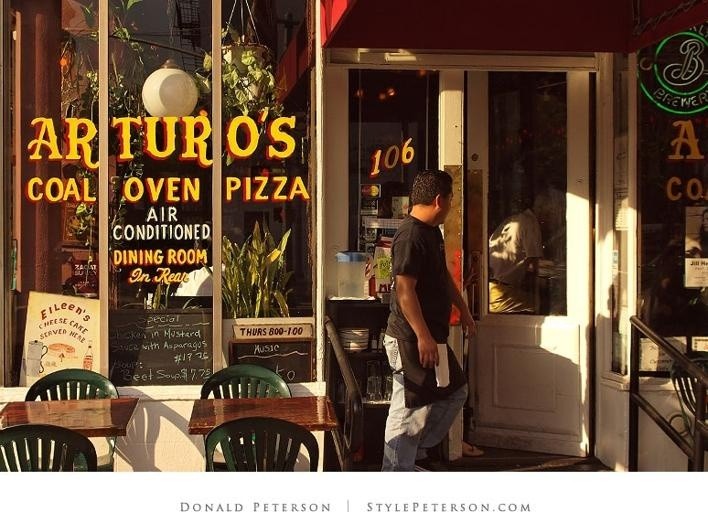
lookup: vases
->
[337,326,370,353]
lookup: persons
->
[460,266,487,455]
[381,169,479,471]
[487,185,567,316]
[172,223,229,298]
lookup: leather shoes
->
[462,446,484,456]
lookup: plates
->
[337,327,370,353]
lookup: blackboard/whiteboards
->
[229,337,317,384]
[109,308,213,387]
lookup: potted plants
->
[194,0,287,117]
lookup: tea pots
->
[22,339,48,376]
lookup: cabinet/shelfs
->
[322,296,469,471]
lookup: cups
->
[336,251,373,298]
[366,376,382,401]
[383,376,392,401]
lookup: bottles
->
[371,327,385,350]
[83,345,93,371]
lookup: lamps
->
[142,1,199,123]
